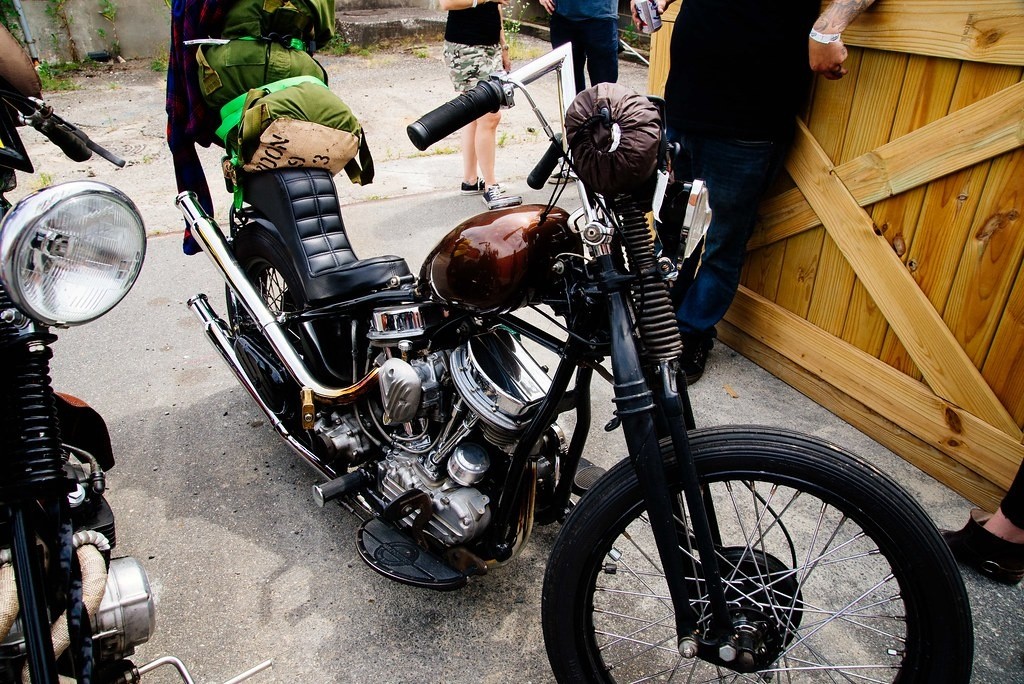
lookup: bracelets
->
[502,44,509,50]
[809,27,841,44]
[472,0,478,8]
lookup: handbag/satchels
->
[536,81,662,228]
[196,0,375,210]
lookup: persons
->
[938,458,1024,586]
[628,0,872,385]
[539,0,619,185]
[438,0,523,210]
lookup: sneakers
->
[460,176,487,196]
[679,326,718,386]
[481,182,522,208]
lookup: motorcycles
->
[0,82,276,684]
[175,37,977,684]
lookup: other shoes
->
[937,507,1024,587]
[548,170,575,184]
